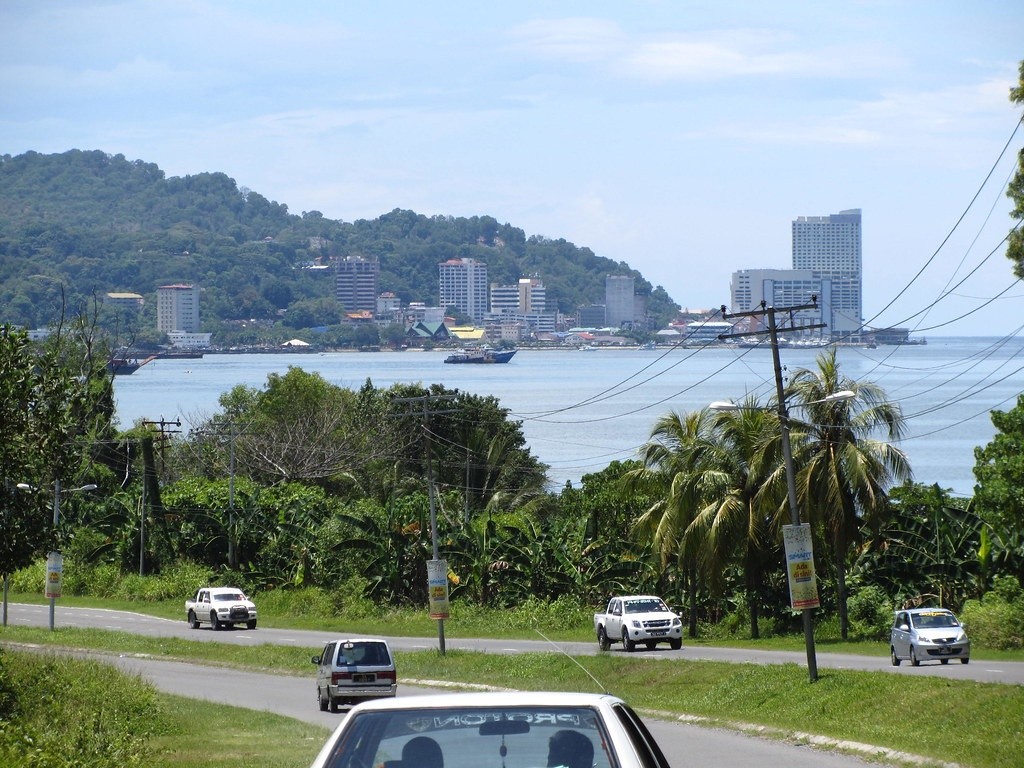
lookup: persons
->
[547,730,594,768]
[401,736,443,768]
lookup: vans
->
[311,639,396,712]
[890,608,971,666]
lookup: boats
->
[109,356,157,375]
[444,349,521,363]
[868,341,876,349]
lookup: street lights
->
[16,482,98,631]
[708,390,856,683]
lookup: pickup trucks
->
[186,587,257,630]
[594,595,682,651]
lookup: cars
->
[308,691,669,768]
